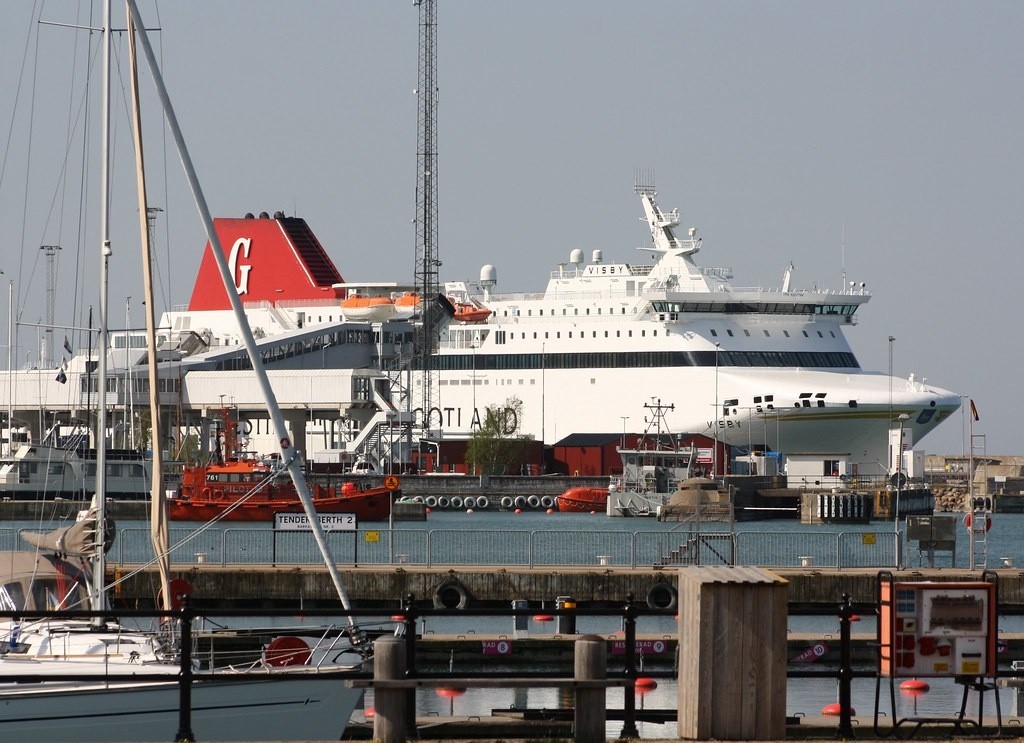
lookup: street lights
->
[713,340,720,476]
[469,343,475,471]
[890,412,911,568]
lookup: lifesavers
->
[413,495,424,505]
[637,580,680,616]
[542,495,554,509]
[476,495,491,510]
[515,494,526,511]
[396,494,412,503]
[197,486,214,501]
[965,512,995,536]
[211,488,227,502]
[500,494,515,511]
[555,495,564,508]
[438,495,450,510]
[527,494,540,509]
[425,494,438,509]
[450,495,464,509]
[462,495,476,510]
[426,580,472,612]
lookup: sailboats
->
[0,1,374,741]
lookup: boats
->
[452,302,493,321]
[170,394,401,523]
[0,167,970,489]
[607,397,707,517]
[0,276,194,499]
